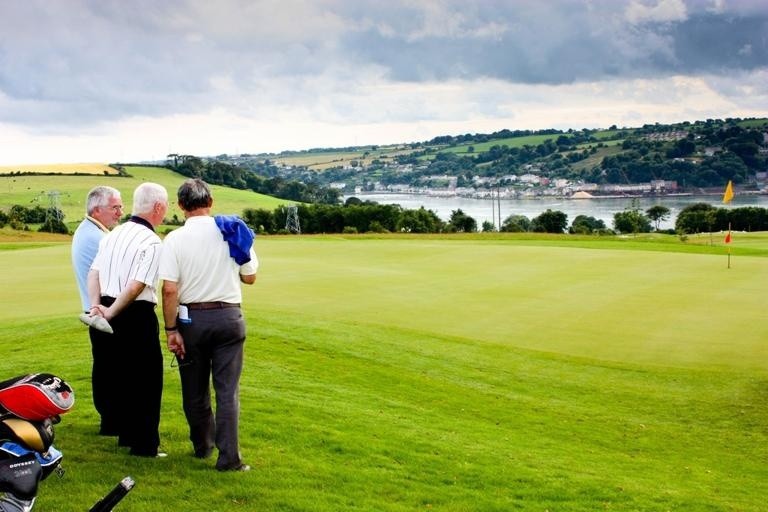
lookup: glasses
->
[171,351,194,368]
[105,206,124,211]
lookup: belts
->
[189,302,240,309]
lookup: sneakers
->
[227,464,251,471]
[155,450,167,457]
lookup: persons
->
[160,178,259,472]
[70,186,126,438]
[86,181,173,459]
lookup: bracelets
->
[164,325,177,331]
